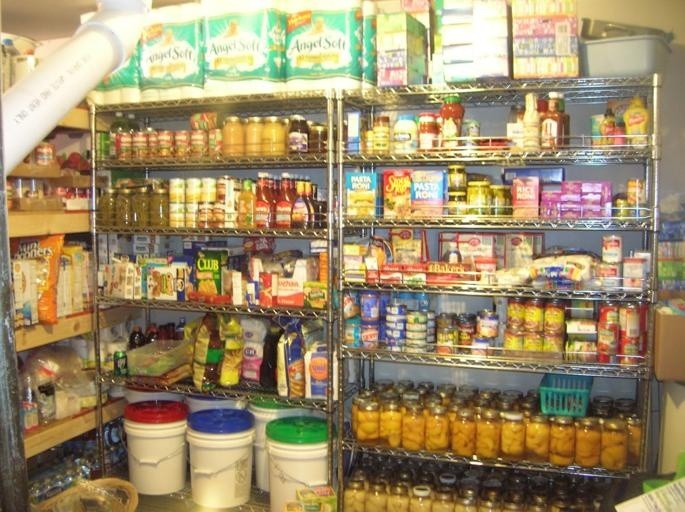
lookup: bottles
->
[366,89,649,151]
[128,318,187,351]
[109,109,140,158]
[238,171,321,229]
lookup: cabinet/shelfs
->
[335,72,667,511]
[0,90,141,466]
[80,86,363,511]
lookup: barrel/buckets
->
[184,393,248,414]
[124,387,186,404]
[246,396,322,493]
[123,398,191,497]
[264,415,337,512]
[186,408,255,509]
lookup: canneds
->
[626,178,644,205]
[7,142,99,200]
[440,96,465,148]
[564,299,598,362]
[115,128,223,160]
[344,291,389,349]
[98,185,170,228]
[345,455,606,512]
[462,119,480,146]
[436,308,500,356]
[190,112,224,131]
[169,175,241,229]
[350,381,642,469]
[506,105,525,154]
[597,301,647,367]
[502,296,566,355]
[418,113,443,149]
[114,350,128,378]
[447,164,510,216]
[222,115,337,157]
[595,262,622,288]
[341,234,651,512]
[385,291,435,353]
[601,236,625,263]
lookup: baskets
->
[539,374,594,417]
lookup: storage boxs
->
[582,32,675,76]
[651,285,684,388]
[648,375,685,479]
